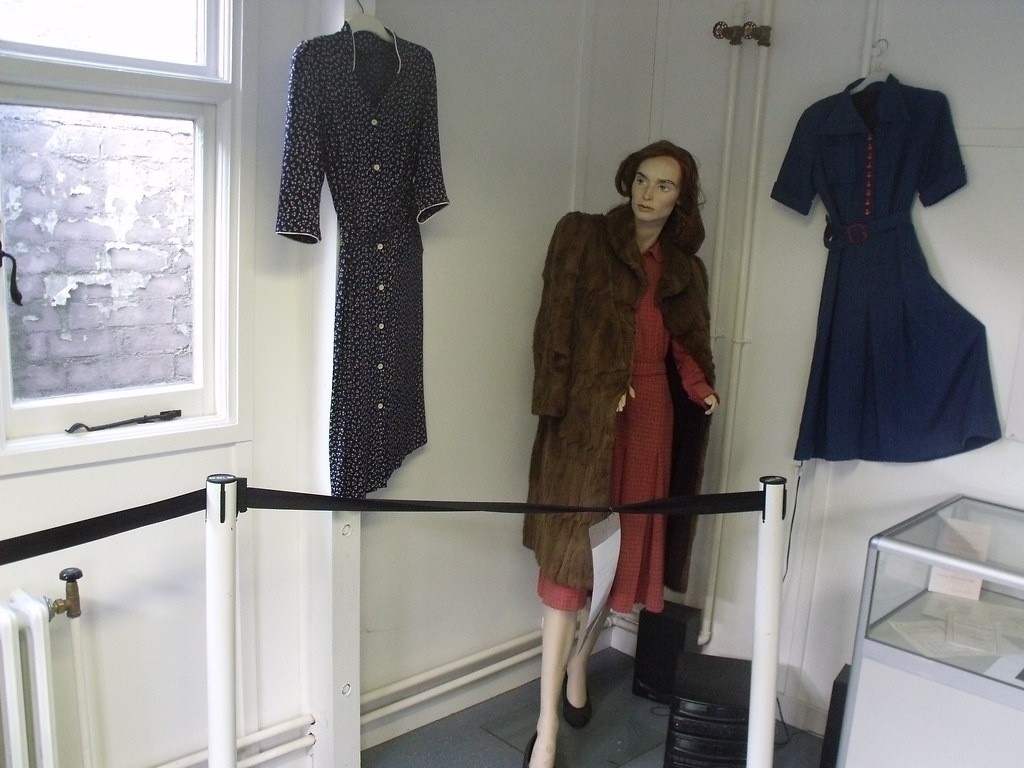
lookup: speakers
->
[632,601,702,704]
[663,652,751,768]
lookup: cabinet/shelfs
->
[838,494,1023,768]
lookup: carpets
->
[480,657,759,768]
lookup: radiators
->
[0,564,80,768]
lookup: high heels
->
[523,731,537,768]
[562,670,593,729]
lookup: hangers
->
[848,39,892,94]
[346,0,395,44]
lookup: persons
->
[523,138,720,768]
